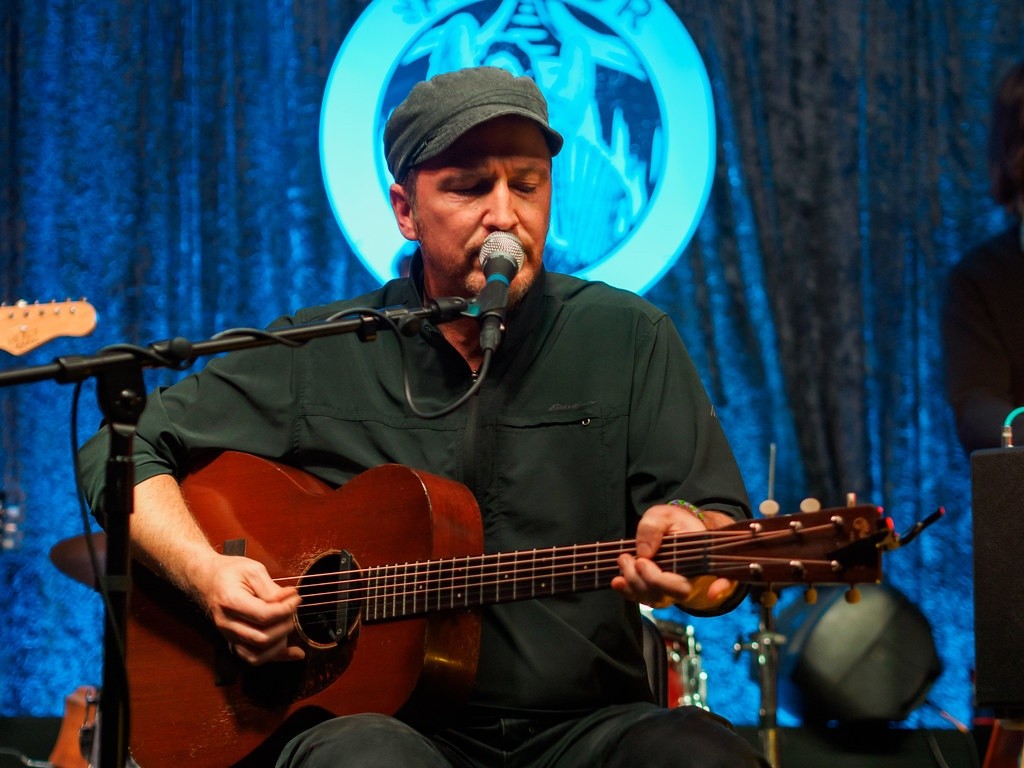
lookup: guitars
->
[0,295,100,359]
[123,447,946,768]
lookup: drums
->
[654,618,705,711]
[772,580,939,725]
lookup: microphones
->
[478,231,524,351]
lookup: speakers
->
[970,447,1024,722]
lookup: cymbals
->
[50,530,109,593]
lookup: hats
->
[384,66,564,185]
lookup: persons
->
[942,60,1024,455]
[71,65,773,768]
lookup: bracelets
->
[669,499,713,529]
